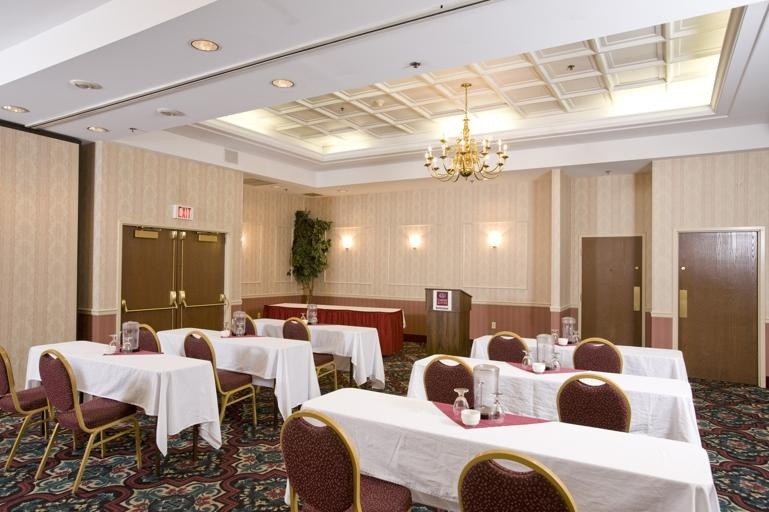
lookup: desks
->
[27,338,223,468]
[159,326,322,432]
[300,385,734,510]
[473,330,693,380]
[404,352,713,440]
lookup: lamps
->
[424,81,510,186]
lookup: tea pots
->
[232,311,247,337]
[561,317,576,344]
[306,303,317,325]
[473,364,499,420]
[536,333,554,368]
[121,320,139,352]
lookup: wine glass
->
[300,312,307,320]
[221,321,231,338]
[108,334,120,353]
[549,352,560,370]
[552,329,559,344]
[235,322,245,337]
[572,330,580,345]
[453,388,469,420]
[522,349,534,370]
[121,337,132,353]
[488,392,505,424]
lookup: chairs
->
[555,372,633,433]
[573,337,624,376]
[457,453,580,512]
[228,317,258,334]
[0,349,52,473]
[181,330,260,440]
[115,323,161,355]
[278,411,411,512]
[280,317,339,394]
[419,356,474,407]
[486,329,529,364]
[35,351,143,495]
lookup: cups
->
[461,410,480,426]
[531,362,545,373]
[557,337,568,346]
